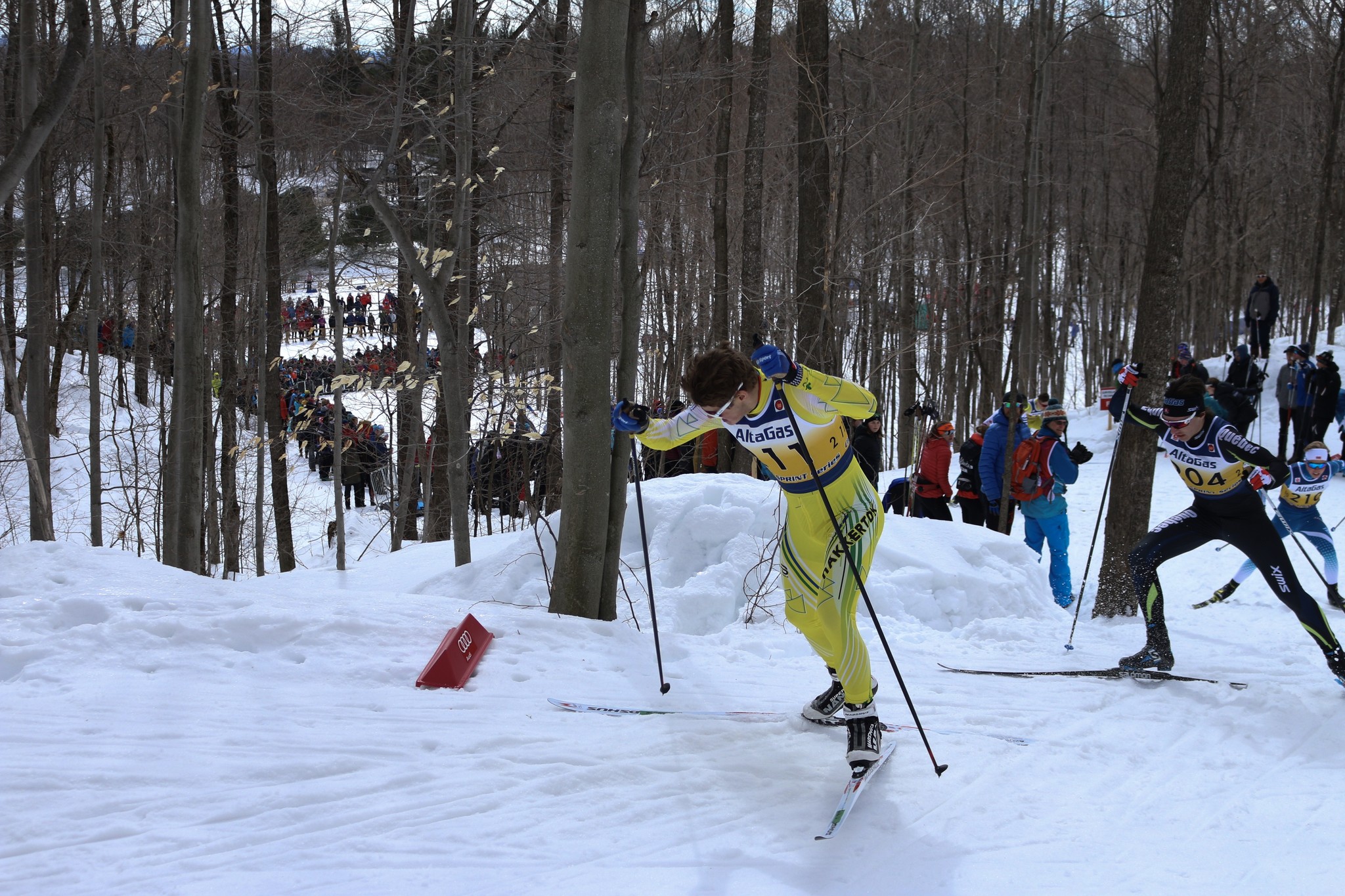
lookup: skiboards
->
[1191,593,1345,610]
[545,691,1030,841]
[934,657,1345,690]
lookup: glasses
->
[1053,419,1068,427]
[691,380,743,419]
[872,413,881,418]
[939,429,957,434]
[1177,346,1190,350]
[1204,383,1213,389]
[1159,410,1196,428]
[1178,350,1191,362]
[1294,349,1300,353]
[1003,401,1022,408]
[1306,459,1326,468]
[1233,353,1240,358]
[1256,274,1266,278]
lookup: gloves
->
[945,495,950,503]
[1117,365,1138,386]
[1269,317,1275,325]
[1249,465,1272,491]
[988,497,1003,513]
[612,400,650,434]
[1071,442,1094,463]
[1244,319,1249,326]
[751,345,798,384]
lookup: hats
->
[1294,343,1312,357]
[865,411,882,426]
[1317,349,1335,364]
[1255,269,1268,275]
[1005,391,1024,410]
[1283,346,1296,353]
[1042,398,1068,433]
[1179,342,1190,349]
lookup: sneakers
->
[1119,645,1177,673]
[1325,642,1345,679]
[1327,585,1345,606]
[1213,579,1239,600]
[807,676,881,729]
[841,699,886,766]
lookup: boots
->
[1275,444,1287,462]
[1287,444,1304,466]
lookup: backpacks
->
[1214,380,1258,425]
[1010,431,1059,500]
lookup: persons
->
[948,407,1000,528]
[1242,269,1280,360]
[881,477,923,519]
[819,275,1086,348]
[1215,441,1345,615]
[7,265,778,520]
[1011,399,1094,608]
[1110,341,1345,477]
[977,390,1032,536]
[841,414,863,441]
[1108,361,1345,691]
[610,342,886,763]
[1021,392,1051,435]
[853,414,886,493]
[911,420,955,522]
